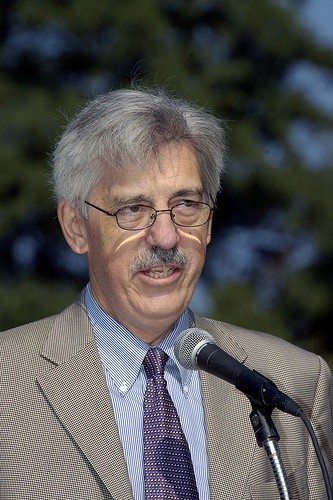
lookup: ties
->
[142,346,200,500]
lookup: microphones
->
[174,327,303,417]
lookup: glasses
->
[77,192,217,231]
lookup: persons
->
[0,85,333,500]
